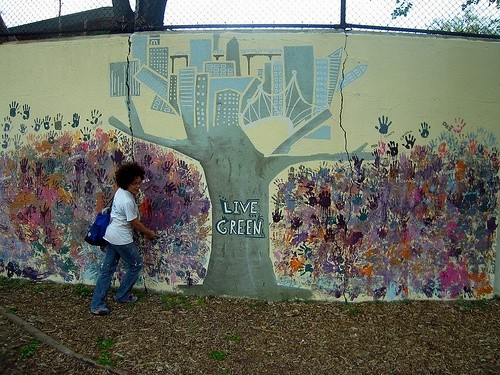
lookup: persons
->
[90,162,161,315]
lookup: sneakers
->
[93,305,111,315]
[119,296,139,303]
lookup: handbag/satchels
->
[85,196,115,247]
